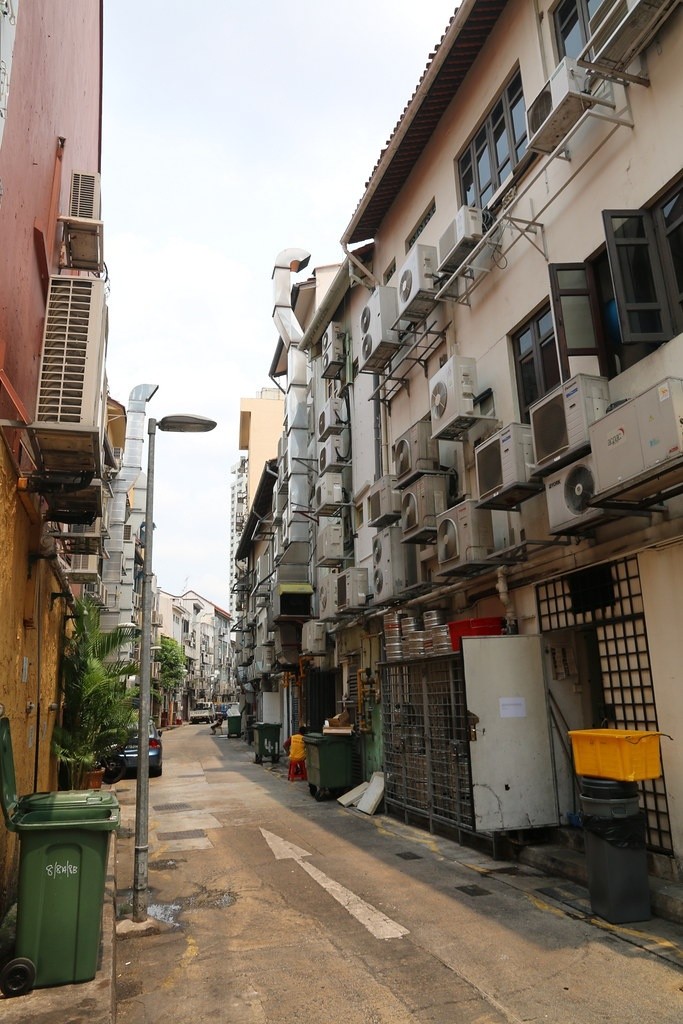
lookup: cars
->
[101,718,164,778]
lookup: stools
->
[288,760,306,781]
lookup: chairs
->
[214,723,223,735]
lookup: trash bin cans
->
[576,774,653,926]
[250,721,282,764]
[226,714,242,740]
[301,732,355,803]
[0,786,121,998]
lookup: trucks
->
[189,700,217,724]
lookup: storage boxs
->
[567,728,662,782]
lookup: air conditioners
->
[32,171,164,675]
[398,244,439,320]
[395,419,438,480]
[371,525,406,601]
[539,453,632,535]
[527,372,613,476]
[335,566,365,614]
[524,56,590,154]
[400,474,448,541]
[428,355,481,440]
[586,376,679,500]
[362,474,402,528]
[588,0,666,65]
[359,286,401,369]
[435,206,484,275]
[435,499,495,577]
[237,322,344,672]
[474,422,545,506]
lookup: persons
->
[211,715,223,735]
[283,725,309,775]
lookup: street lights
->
[132,411,218,925]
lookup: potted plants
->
[52,588,140,791]
